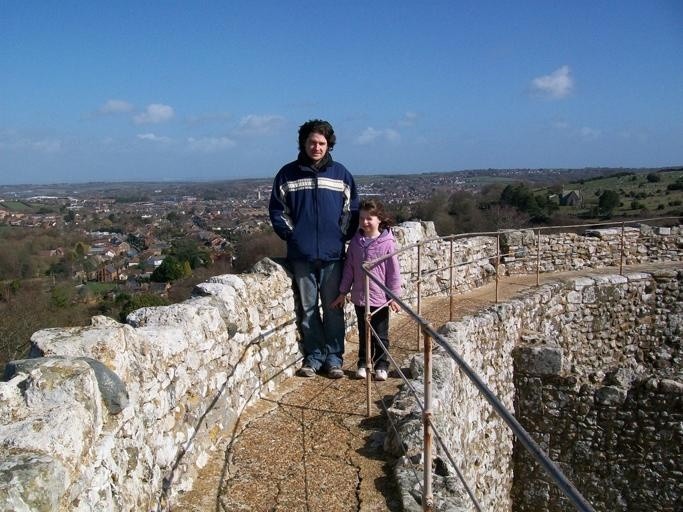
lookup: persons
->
[267,117,362,379]
[328,196,404,382]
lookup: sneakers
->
[326,362,344,379]
[354,366,367,378]
[374,369,389,381]
[298,361,320,376]
